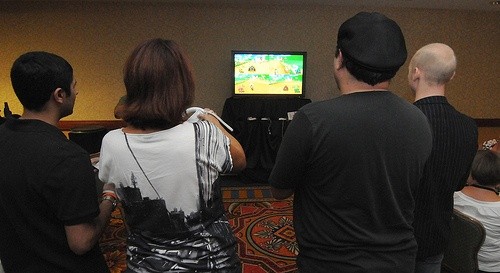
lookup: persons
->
[268,12,432,273]
[454,148,500,273]
[0,52,117,273]
[407,43,479,273]
[98,39,246,273]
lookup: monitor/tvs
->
[230,50,307,98]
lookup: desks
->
[222,96,314,181]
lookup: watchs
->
[100,195,117,211]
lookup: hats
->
[337,11,407,72]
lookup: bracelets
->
[101,191,118,199]
